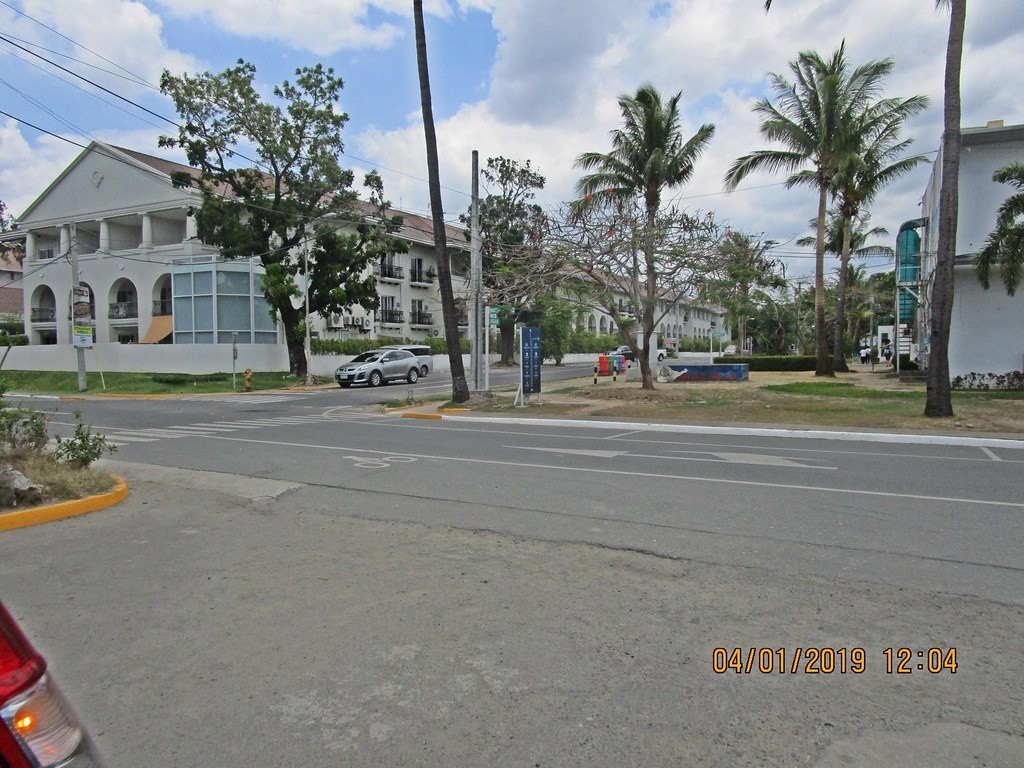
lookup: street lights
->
[304,212,338,388]
[744,317,755,350]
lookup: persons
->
[859,347,866,364]
[883,339,896,367]
[866,346,871,363]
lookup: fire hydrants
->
[243,367,254,392]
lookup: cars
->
[723,345,739,357]
[335,348,422,389]
[602,345,638,362]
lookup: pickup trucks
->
[656,348,668,362]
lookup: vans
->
[373,344,433,378]
